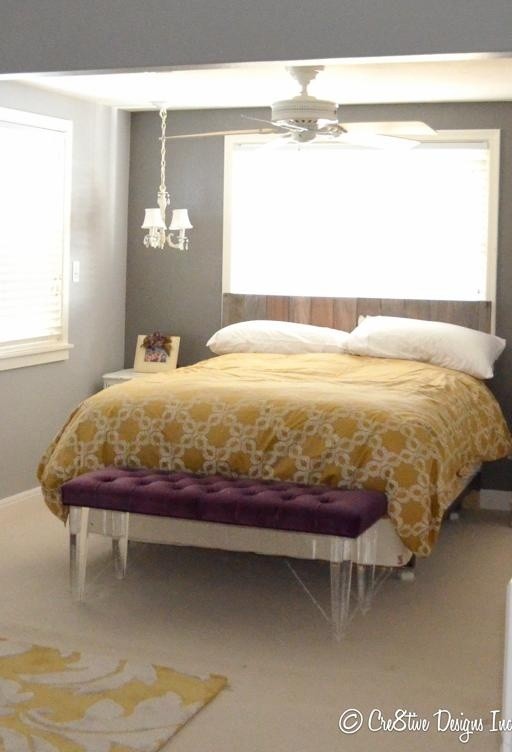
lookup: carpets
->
[0,631,228,750]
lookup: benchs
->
[60,464,388,641]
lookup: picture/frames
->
[132,333,180,373]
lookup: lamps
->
[137,105,195,255]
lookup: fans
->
[155,62,440,158]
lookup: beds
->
[37,292,499,579]
